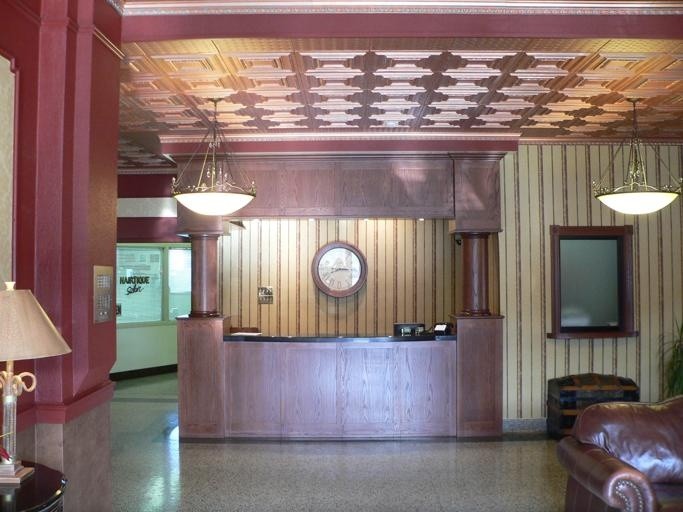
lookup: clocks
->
[312,241,369,298]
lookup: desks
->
[0,459,68,512]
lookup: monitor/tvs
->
[394,323,425,336]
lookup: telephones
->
[435,323,453,335]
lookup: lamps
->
[171,96,257,218]
[0,281,74,483]
[592,96,683,216]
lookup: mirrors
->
[545,225,639,339]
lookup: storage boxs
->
[546,373,640,441]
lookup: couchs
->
[557,394,683,512]
